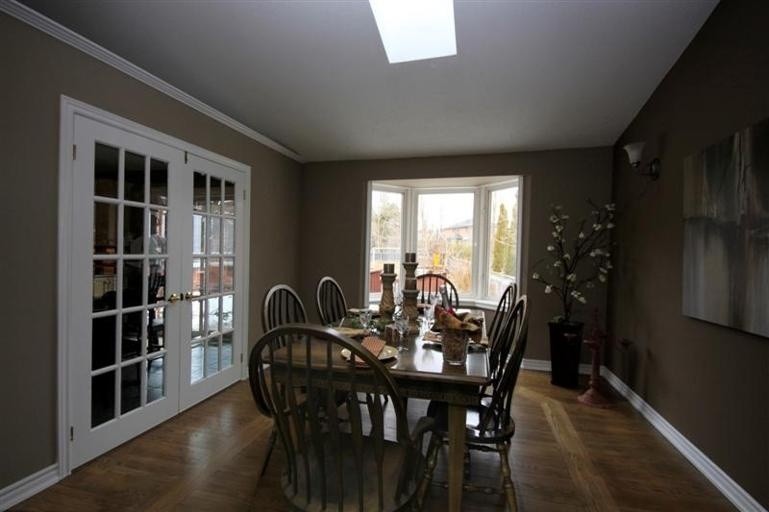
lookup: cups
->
[395,316,410,336]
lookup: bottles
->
[440,285,460,320]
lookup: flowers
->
[531,199,620,318]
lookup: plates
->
[341,343,398,364]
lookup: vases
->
[546,318,585,391]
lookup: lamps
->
[624,140,663,183]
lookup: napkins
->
[346,336,387,364]
[431,305,484,343]
[424,332,488,348]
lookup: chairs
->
[254,283,338,491]
[423,294,531,511]
[316,276,388,405]
[248,324,442,512]
[416,271,460,313]
[427,281,517,478]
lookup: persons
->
[126,211,166,382]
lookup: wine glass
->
[360,312,373,336]
